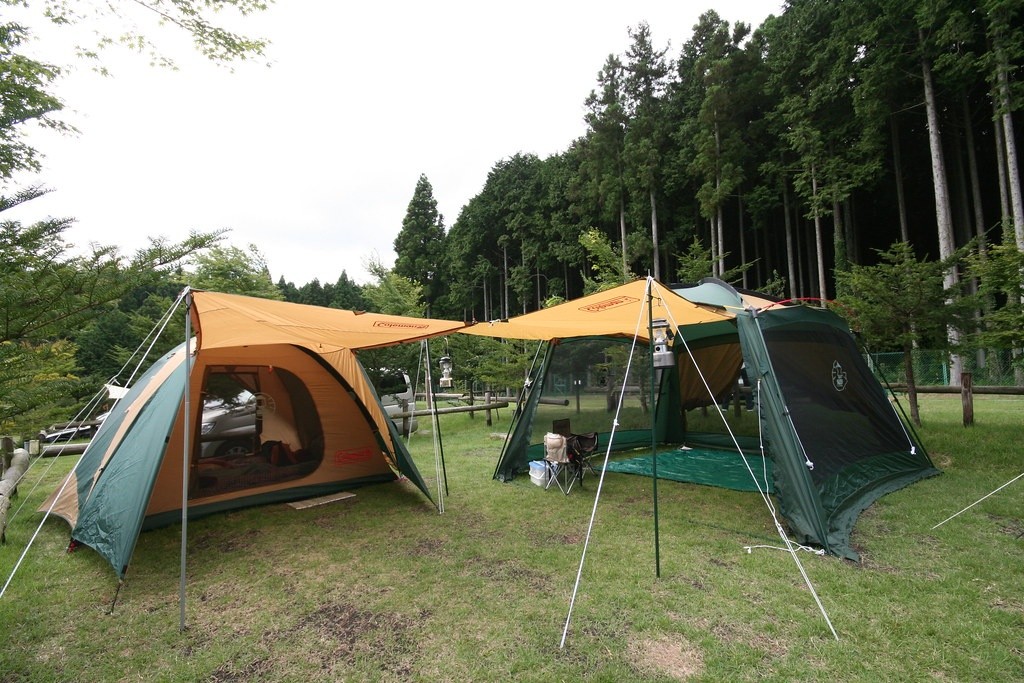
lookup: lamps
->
[438,338,454,388]
[646,298,676,369]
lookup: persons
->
[95,402,109,416]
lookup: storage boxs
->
[529,461,550,486]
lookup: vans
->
[198,367,419,459]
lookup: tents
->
[455,272,946,564]
[37,290,478,614]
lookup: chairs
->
[553,418,598,480]
[543,432,582,495]
